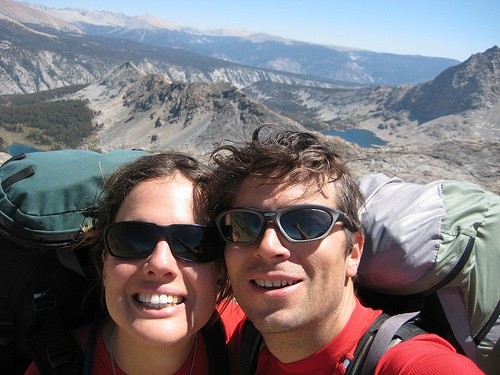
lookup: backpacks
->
[0,142,229,375]
[347,172,500,374]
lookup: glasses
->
[99,220,224,263]
[212,202,357,246]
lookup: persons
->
[177,238,210,261]
[202,121,487,375]
[225,214,237,241]
[24,153,252,375]
[295,224,308,239]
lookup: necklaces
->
[110,324,197,375]
